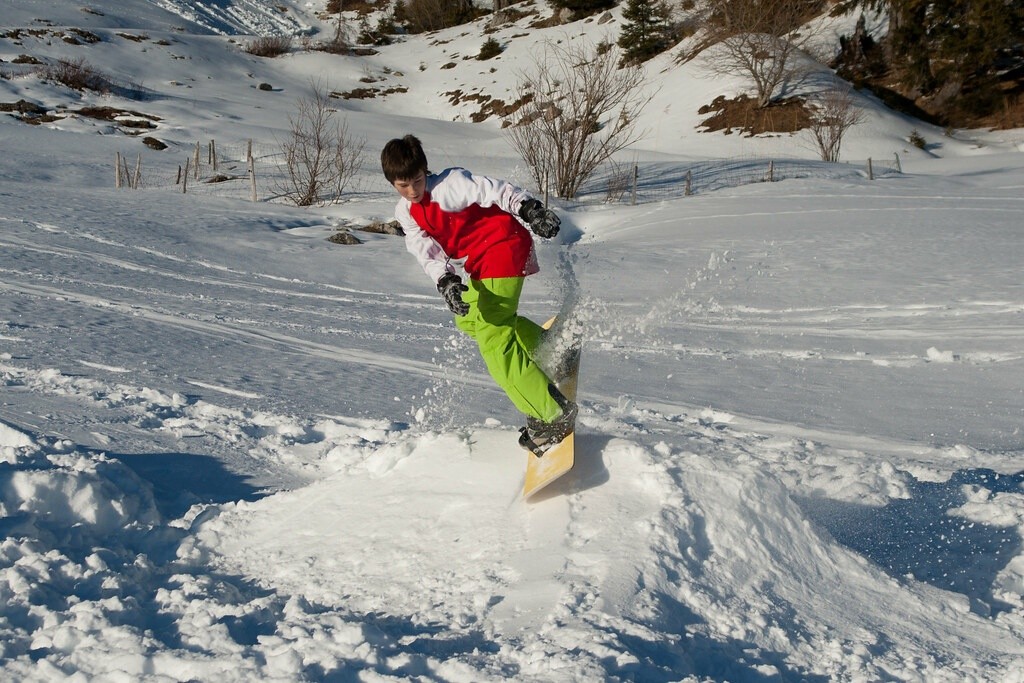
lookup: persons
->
[380,133,578,452]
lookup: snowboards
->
[523,315,582,499]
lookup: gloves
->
[437,273,470,317]
[518,199,561,238]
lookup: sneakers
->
[518,403,578,450]
